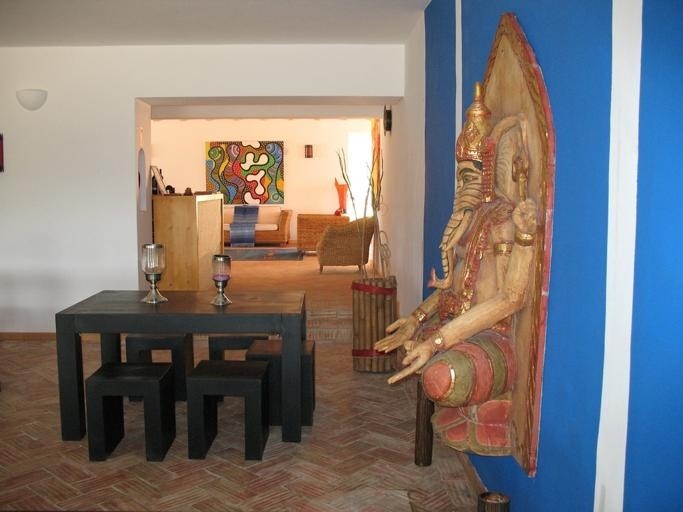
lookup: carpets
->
[224,249,303,261]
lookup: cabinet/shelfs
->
[153,191,224,290]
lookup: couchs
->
[224,206,293,247]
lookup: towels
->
[230,205,258,248]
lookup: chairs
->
[297,214,349,254]
[316,216,375,274]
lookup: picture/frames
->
[151,165,168,195]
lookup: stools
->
[86,363,177,463]
[186,359,270,461]
[246,341,316,427]
[208,334,268,402]
[125,331,193,402]
